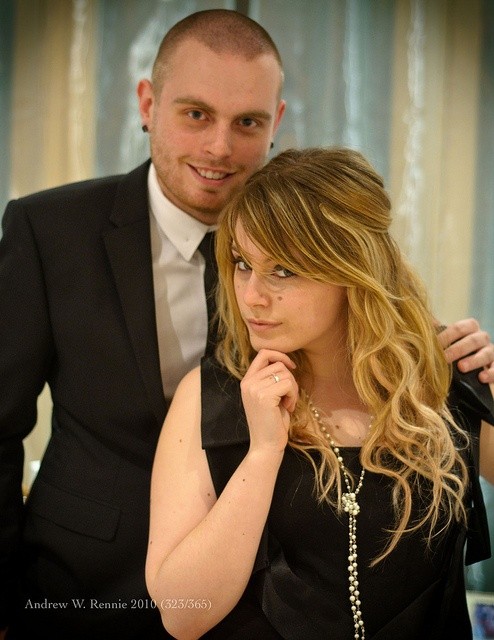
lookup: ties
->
[197,230,226,356]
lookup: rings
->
[272,374,278,382]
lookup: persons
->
[142,147,492,640]
[0,8,493,640]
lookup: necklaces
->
[301,389,374,640]
